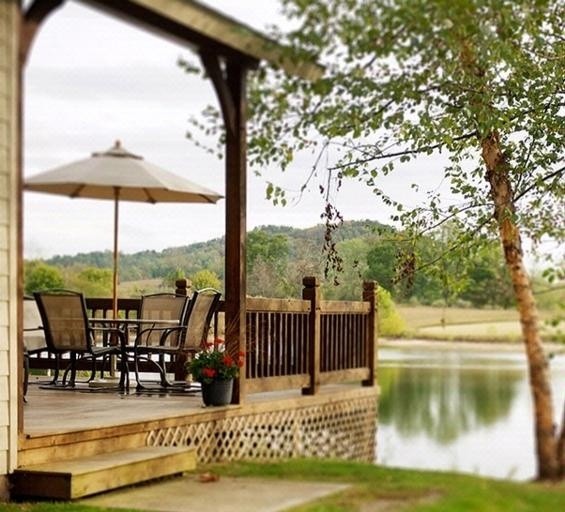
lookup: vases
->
[200,380,234,405]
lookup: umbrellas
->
[22,139,224,378]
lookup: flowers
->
[183,320,248,380]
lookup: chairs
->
[21,287,220,396]
